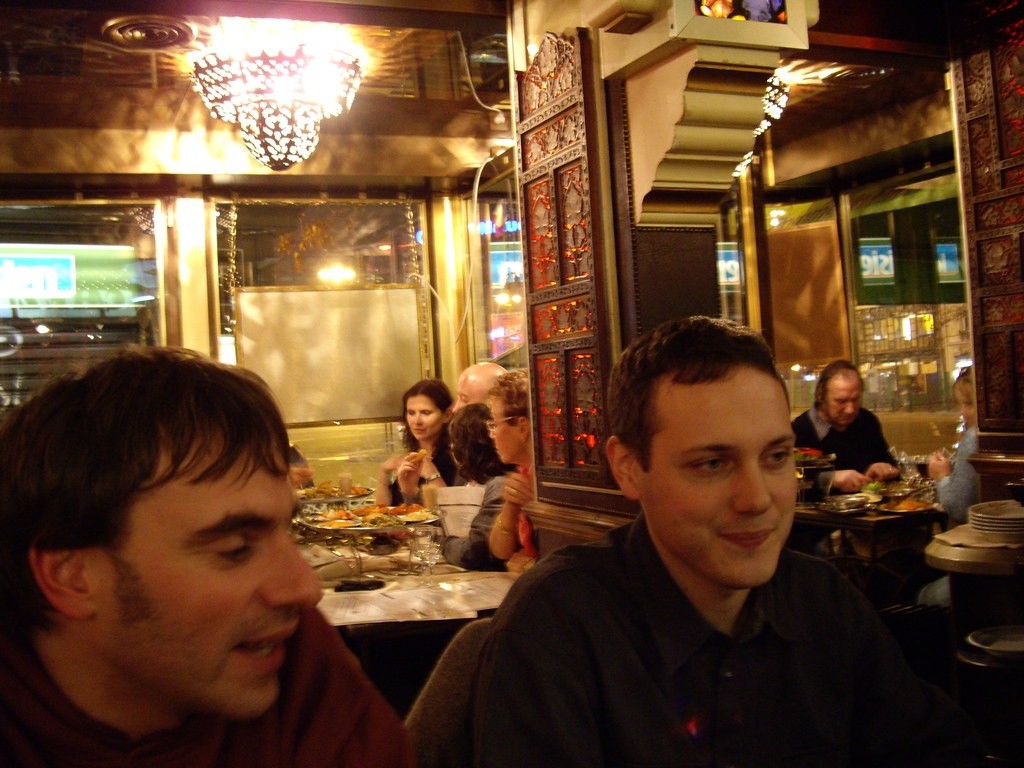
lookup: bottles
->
[366,543,392,555]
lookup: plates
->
[969,499,1024,542]
[879,502,938,512]
[301,511,362,528]
[361,506,447,521]
[835,504,869,513]
[296,487,375,502]
[853,493,882,502]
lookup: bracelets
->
[497,515,517,534]
[425,472,442,483]
[379,479,392,487]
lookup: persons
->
[468,316,984,768]
[287,362,539,574]
[917,368,982,609]
[790,361,902,576]
[0,346,414,768]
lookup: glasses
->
[486,415,521,429]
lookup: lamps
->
[188,17,371,171]
[732,75,790,180]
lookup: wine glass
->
[415,524,444,574]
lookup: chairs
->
[400,618,496,768]
[826,554,918,599]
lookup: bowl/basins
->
[825,495,869,509]
[813,502,832,511]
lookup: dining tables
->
[299,540,524,668]
[793,501,949,574]
